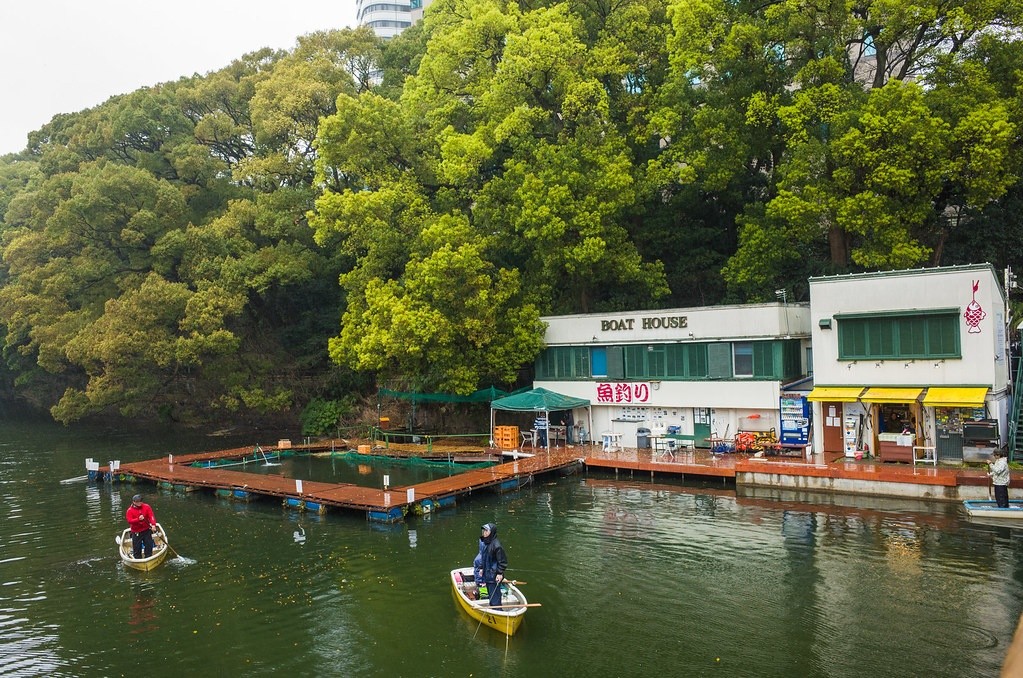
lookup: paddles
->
[501,580,528,585]
[471,602,542,610]
[142,515,185,560]
[987,463,995,501]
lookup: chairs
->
[520,431,534,450]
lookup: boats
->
[115,521,169,573]
[963,497,1023,519]
[451,566,527,637]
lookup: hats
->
[990,448,1004,455]
[482,524,491,531]
[133,495,143,501]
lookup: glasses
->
[134,499,142,502]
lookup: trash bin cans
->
[636,428,651,449]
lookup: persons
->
[126,495,156,559]
[534,412,551,449]
[987,449,1010,508]
[474,523,507,612]
[561,409,574,448]
[886,413,902,433]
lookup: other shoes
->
[544,446,547,449]
[540,445,543,448]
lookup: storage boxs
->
[494,426,519,448]
[358,445,370,454]
[279,439,291,447]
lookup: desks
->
[704,437,723,461]
[878,433,916,464]
[602,433,624,454]
[661,434,695,451]
[530,429,540,447]
[550,429,565,450]
[762,443,810,458]
[647,435,666,455]
[660,438,676,458]
[913,446,937,466]
[390,427,437,443]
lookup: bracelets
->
[989,462,992,465]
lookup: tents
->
[490,387,593,454]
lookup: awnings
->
[922,387,990,417]
[806,388,864,415]
[860,388,925,417]
